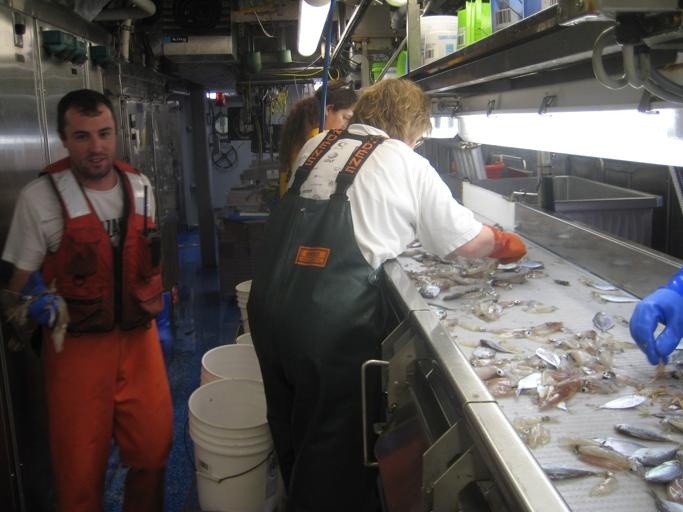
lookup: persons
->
[278,82,356,200]
[0,87,176,512]
[245,77,528,512]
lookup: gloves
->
[21,271,62,331]
[154,291,174,360]
[482,223,528,264]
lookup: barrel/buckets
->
[236,331,256,344]
[235,279,251,334]
[420,14,458,66]
[200,344,263,385]
[187,378,281,511]
[370,62,396,84]
